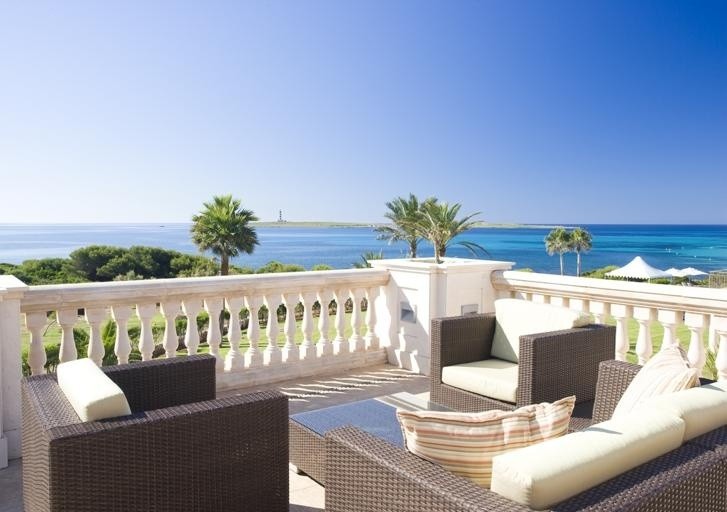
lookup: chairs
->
[21,353,288,512]
[429,313,616,416]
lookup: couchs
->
[326,360,727,512]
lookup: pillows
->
[56,358,131,422]
[492,298,592,364]
